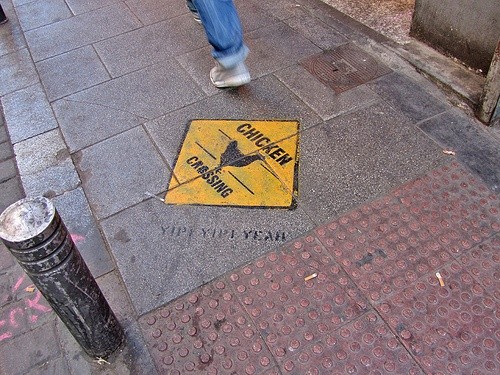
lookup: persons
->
[186,0,251,88]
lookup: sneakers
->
[210,59,251,87]
[191,11,203,25]
